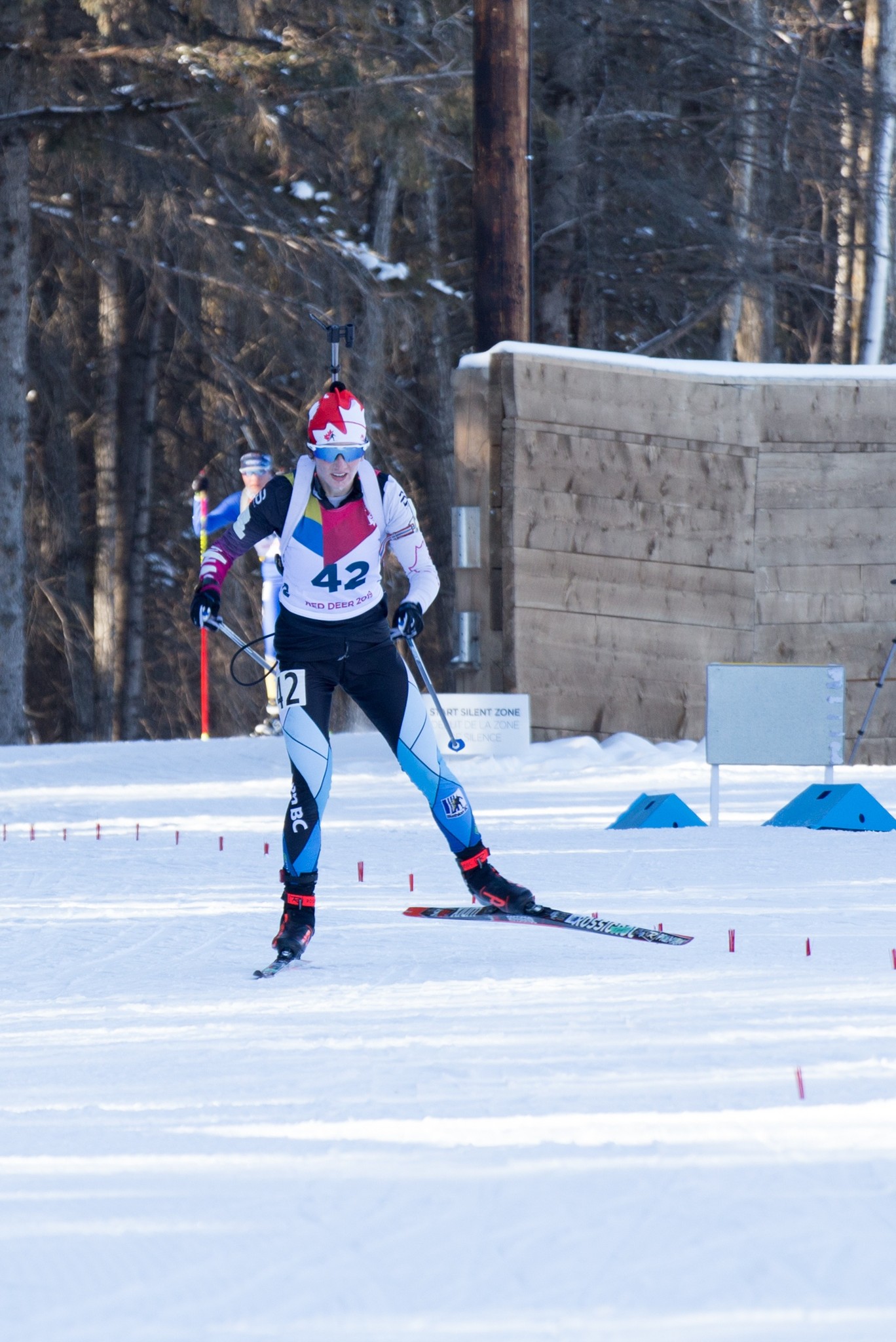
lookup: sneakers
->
[454,840,535,915]
[270,868,318,959]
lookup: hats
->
[239,452,272,473]
[307,381,367,445]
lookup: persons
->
[192,450,283,734]
[190,380,535,960]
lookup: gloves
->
[191,471,209,493]
[190,589,222,633]
[389,602,424,642]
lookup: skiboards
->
[252,903,694,979]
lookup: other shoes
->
[254,714,282,735]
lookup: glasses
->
[241,470,267,476]
[307,439,370,464]
